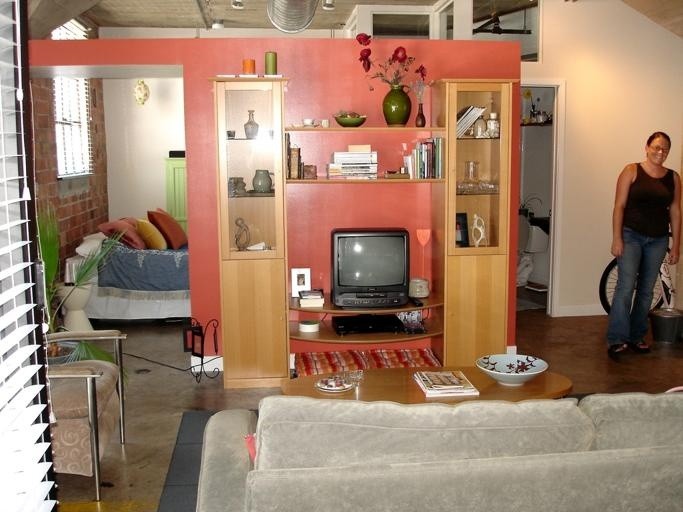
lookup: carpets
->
[158,392,600,511]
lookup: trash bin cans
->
[648,308,682,342]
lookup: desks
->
[57,283,93,331]
[279,366,573,406]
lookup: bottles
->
[536,110,548,124]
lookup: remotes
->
[412,298,423,306]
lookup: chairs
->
[40,327,125,501]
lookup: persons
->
[606,131,683,358]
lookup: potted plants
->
[37,202,131,396]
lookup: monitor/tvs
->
[331,227,410,307]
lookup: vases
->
[650,308,682,346]
[529,105,537,119]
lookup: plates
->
[302,124,319,129]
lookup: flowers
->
[356,33,414,91]
[413,64,428,104]
[521,88,541,107]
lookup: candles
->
[243,59,255,75]
[265,52,277,75]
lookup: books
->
[298,290,325,309]
[413,371,480,399]
[286,135,443,180]
[454,98,488,137]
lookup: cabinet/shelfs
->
[166,157,186,238]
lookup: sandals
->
[633,340,649,354]
[608,344,624,361]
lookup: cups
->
[320,119,330,129]
[263,52,277,75]
[241,58,256,76]
[302,118,315,125]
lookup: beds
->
[62,247,192,322]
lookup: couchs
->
[195,386,683,511]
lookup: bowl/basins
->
[333,115,366,128]
[473,353,550,388]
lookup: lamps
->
[134,80,150,106]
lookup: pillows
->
[74,205,187,256]
